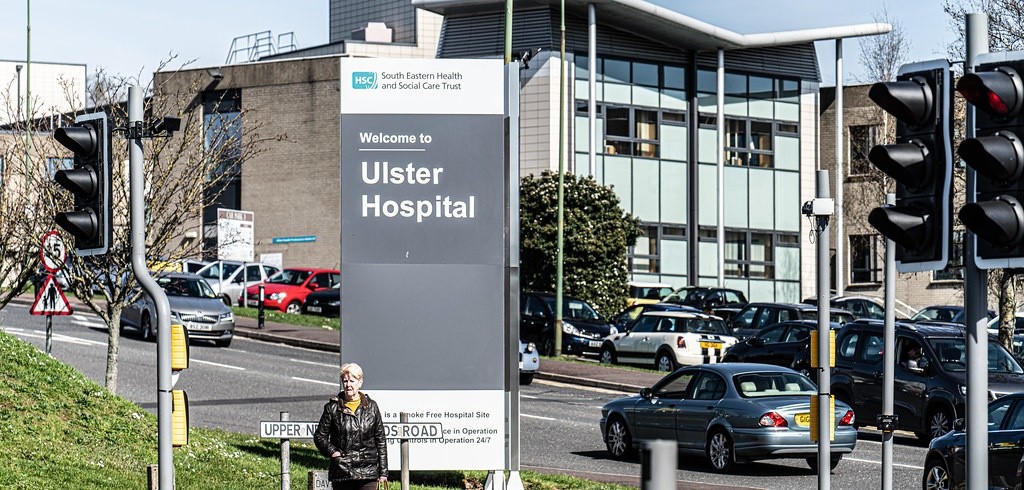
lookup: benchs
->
[741,382,815,395]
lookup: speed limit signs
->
[39,231,66,272]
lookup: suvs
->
[727,295,1024,340]
[830,318,1024,443]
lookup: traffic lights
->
[869,59,953,272]
[956,52,1024,272]
[54,111,113,258]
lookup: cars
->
[598,312,739,372]
[722,319,882,381]
[623,282,678,318]
[518,340,539,385]
[600,362,857,476]
[238,267,341,315]
[302,283,340,318]
[108,272,234,348]
[519,292,618,357]
[659,286,752,322]
[33,256,126,300]
[923,392,1024,490]
[607,304,706,334]
[193,261,288,307]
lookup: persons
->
[313,363,388,490]
[33,272,46,300]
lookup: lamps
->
[213,74,223,81]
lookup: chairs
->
[854,304,862,314]
[698,381,725,400]
[645,288,660,299]
[660,320,673,332]
[935,310,952,321]
[299,272,311,286]
[942,347,965,371]
[796,330,810,341]
